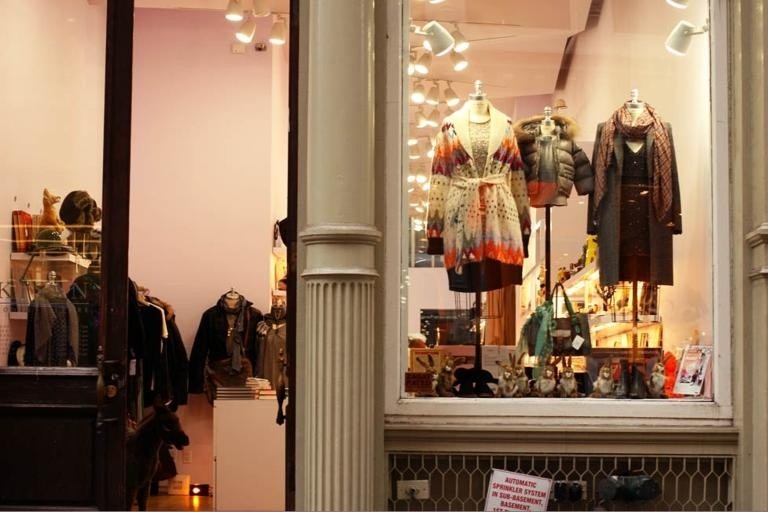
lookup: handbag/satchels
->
[528,283,591,356]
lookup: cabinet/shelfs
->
[555,257,661,339]
[9,251,91,321]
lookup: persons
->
[514,105,595,209]
[584,90,682,287]
[253,298,286,384]
[184,286,264,385]
[419,81,534,296]
[22,271,78,367]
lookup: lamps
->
[664,0,709,56]
[224,0,287,45]
[407,0,471,232]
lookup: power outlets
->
[397,480,430,499]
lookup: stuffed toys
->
[414,351,672,399]
